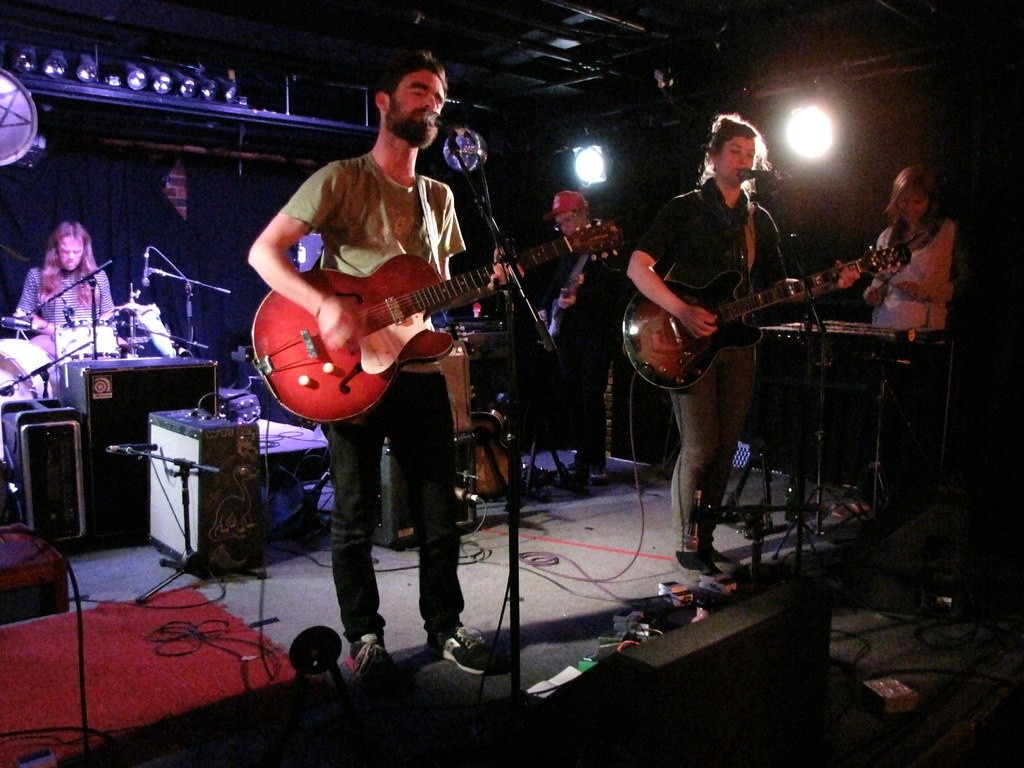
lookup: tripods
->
[772,341,897,558]
[117,447,267,605]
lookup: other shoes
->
[831,497,872,520]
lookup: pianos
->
[755,322,947,351]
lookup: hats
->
[543,190,588,220]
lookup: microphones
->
[423,109,472,138]
[108,443,158,454]
[1,317,43,330]
[142,247,150,287]
[103,311,120,326]
[0,385,15,397]
[454,487,482,501]
[736,167,791,180]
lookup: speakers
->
[58,357,219,550]
[543,490,1024,768]
[148,408,266,564]
[372,340,479,548]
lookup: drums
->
[57,317,117,359]
[1,338,54,402]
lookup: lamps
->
[10,42,243,104]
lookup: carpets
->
[0,588,296,768]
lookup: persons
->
[537,190,627,488]
[243,49,525,684]
[13,220,145,360]
[626,111,861,584]
[862,166,955,333]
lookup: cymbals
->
[0,316,34,331]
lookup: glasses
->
[554,211,579,231]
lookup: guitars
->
[623,241,912,392]
[542,215,599,352]
[251,222,626,424]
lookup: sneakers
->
[344,631,400,687]
[674,550,725,582]
[710,546,746,574]
[557,460,588,483]
[429,621,499,675]
[588,464,607,485]
